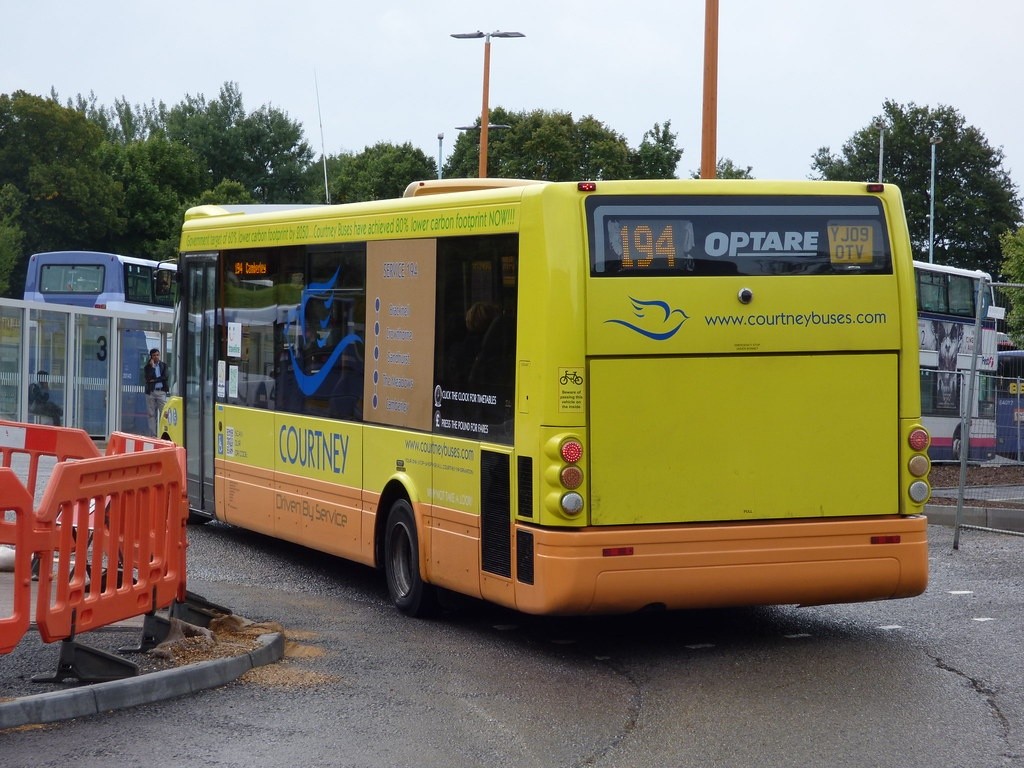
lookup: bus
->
[912,260,1023,460]
[152,178,930,619]
[18,251,273,438]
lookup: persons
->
[28,370,63,427]
[143,348,169,438]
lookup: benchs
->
[0,412,42,424]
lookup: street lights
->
[450,29,526,178]
[438,132,444,179]
[873,120,886,181]
[928,133,944,285]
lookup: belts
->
[154,387,165,391]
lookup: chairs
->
[469,309,514,387]
